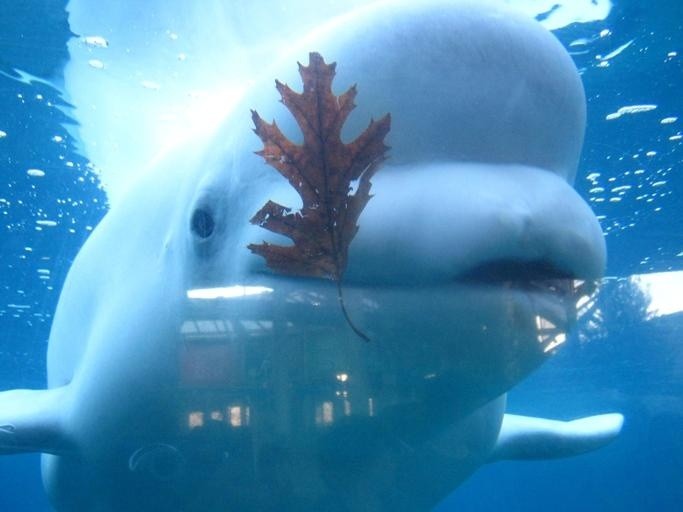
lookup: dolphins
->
[0,0,626,512]
